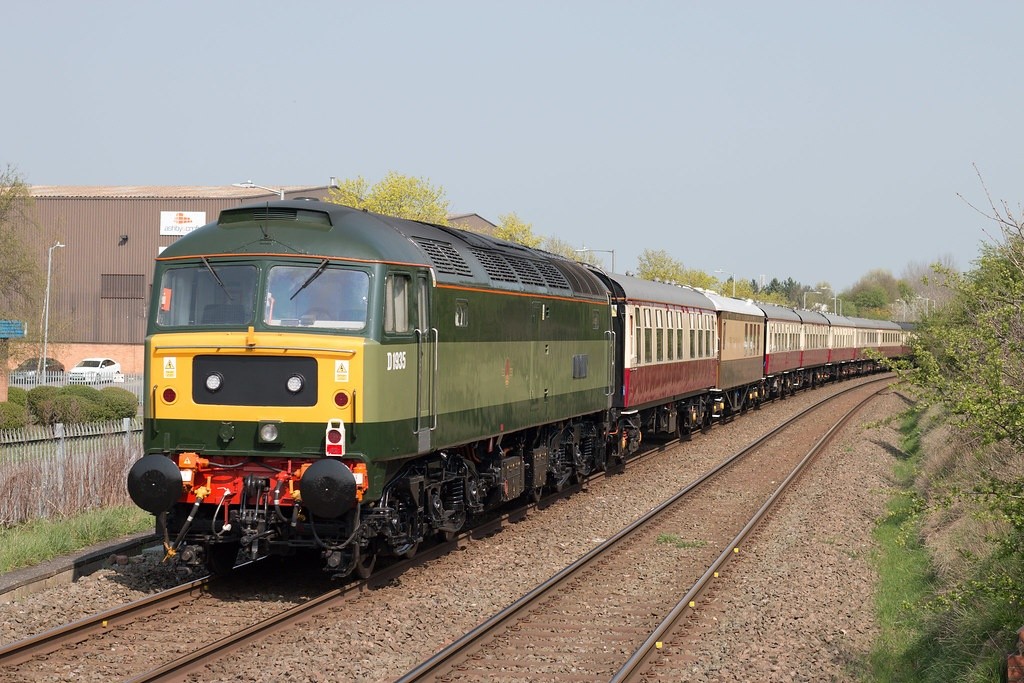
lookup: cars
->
[68,356,122,384]
[10,356,64,383]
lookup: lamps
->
[119,235,128,241]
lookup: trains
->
[124,196,925,594]
